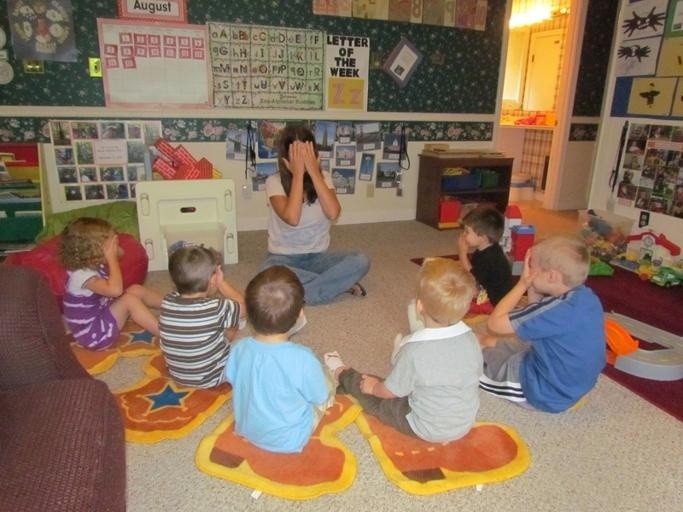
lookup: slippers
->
[348,282,367,297]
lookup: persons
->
[460,207,511,317]
[325,257,483,444]
[256,122,371,304]
[157,246,246,389]
[52,218,166,351]
[224,267,337,454]
[475,236,606,414]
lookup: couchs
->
[35,200,140,244]
[0,265,125,512]
[0,232,148,313]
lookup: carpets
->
[93,199,666,511]
[410,254,683,420]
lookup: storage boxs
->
[478,201,497,211]
[160,224,227,269]
[441,167,481,191]
[481,170,499,188]
[460,200,479,219]
[439,196,461,223]
[576,209,636,262]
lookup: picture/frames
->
[320,157,332,178]
[333,142,357,170]
[330,167,357,195]
[626,76,679,117]
[383,37,424,88]
[118,0,187,22]
[613,36,664,78]
[96,18,214,109]
[251,122,286,161]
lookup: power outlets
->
[22,60,44,74]
[88,57,102,77]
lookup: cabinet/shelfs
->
[416,154,514,231]
